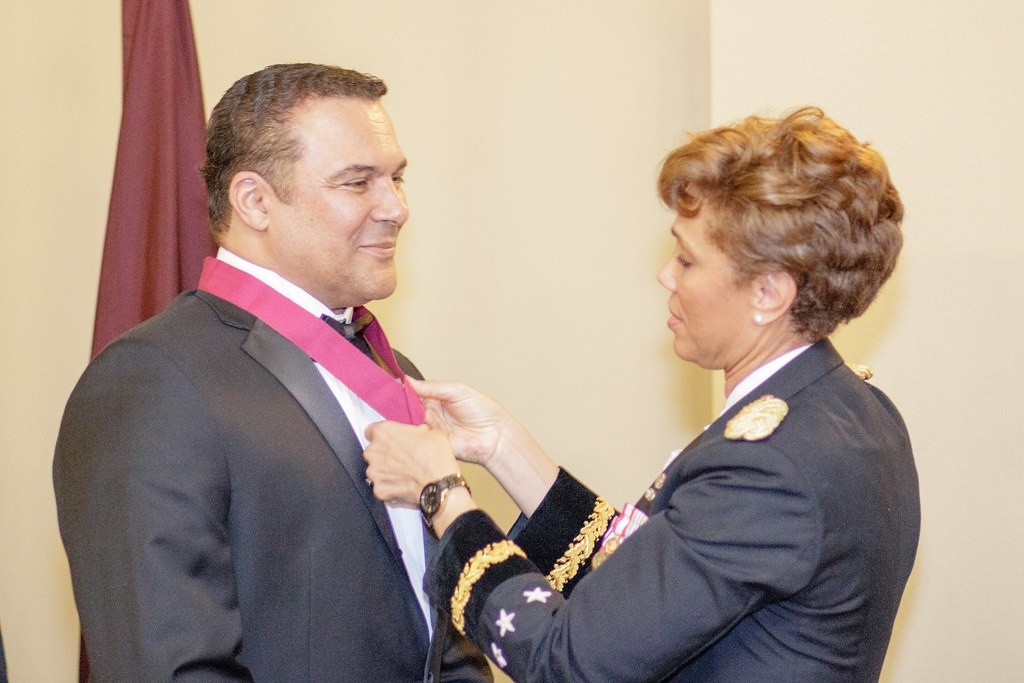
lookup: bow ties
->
[311,313,375,362]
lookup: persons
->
[51,63,494,683]
[362,106,922,683]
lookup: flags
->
[89,0,218,369]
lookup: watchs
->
[418,472,471,542]
